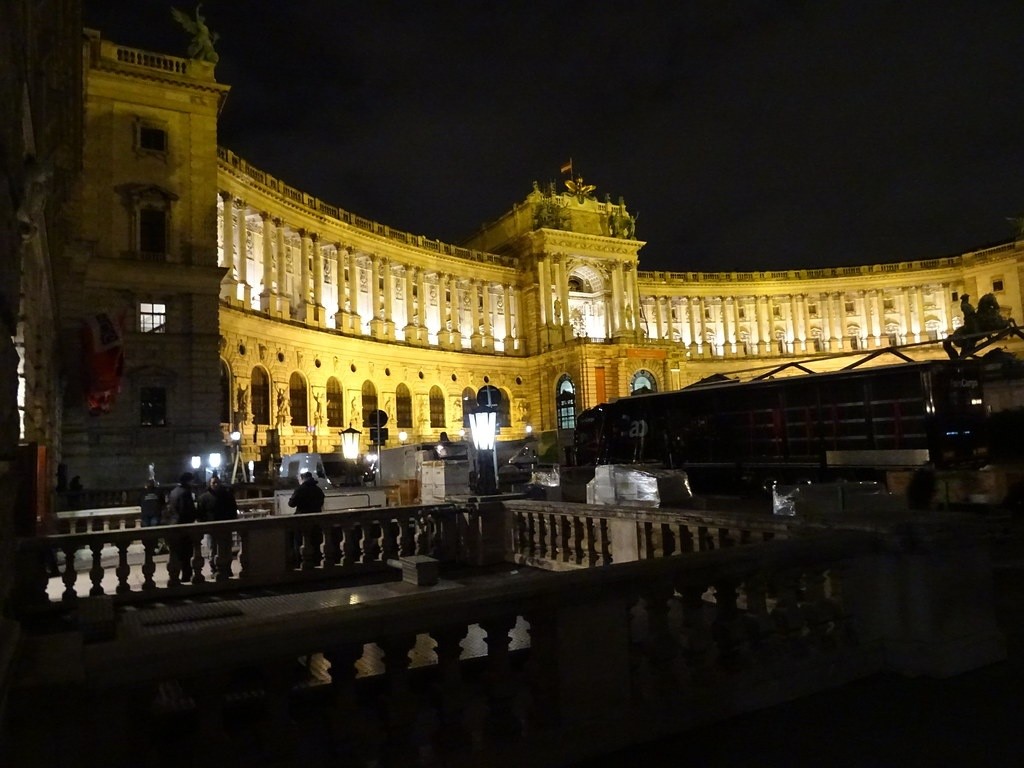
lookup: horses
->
[942,294,1024,360]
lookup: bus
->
[571,329,1024,516]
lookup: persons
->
[961,293,980,334]
[288,471,325,557]
[138,470,238,582]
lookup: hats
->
[301,472,312,477]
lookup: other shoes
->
[180,577,191,582]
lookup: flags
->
[78,300,127,414]
